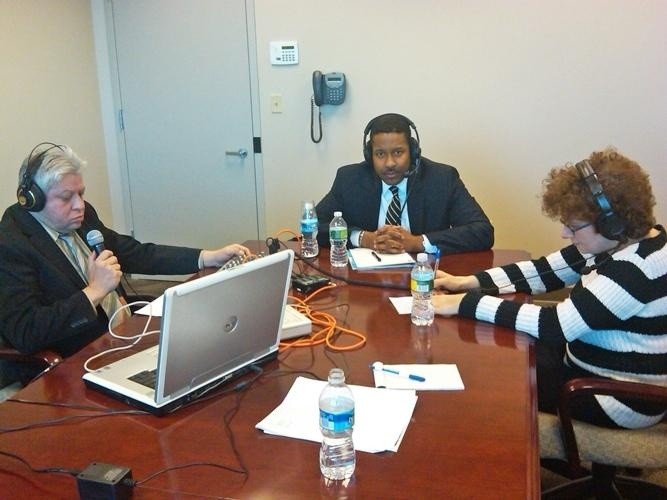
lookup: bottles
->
[320,367,355,480]
[329,210,349,267]
[302,201,319,258]
[410,253,434,326]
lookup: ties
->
[384,185,403,226]
[58,232,132,327]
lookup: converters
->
[75,462,136,496]
[292,273,331,294]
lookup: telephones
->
[310,71,346,143]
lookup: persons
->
[431,153,667,429]
[0,147,252,389]
[312,114,494,256]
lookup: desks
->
[0,239,539,500]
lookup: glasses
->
[559,218,592,234]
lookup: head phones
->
[359,113,421,178]
[567,159,630,277]
[16,140,68,214]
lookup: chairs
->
[540,377,667,499]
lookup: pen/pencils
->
[372,252,381,261]
[434,250,442,279]
[369,366,426,383]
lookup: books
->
[371,359,466,394]
[345,246,417,271]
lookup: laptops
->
[82,248,294,417]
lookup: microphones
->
[87,229,125,296]
